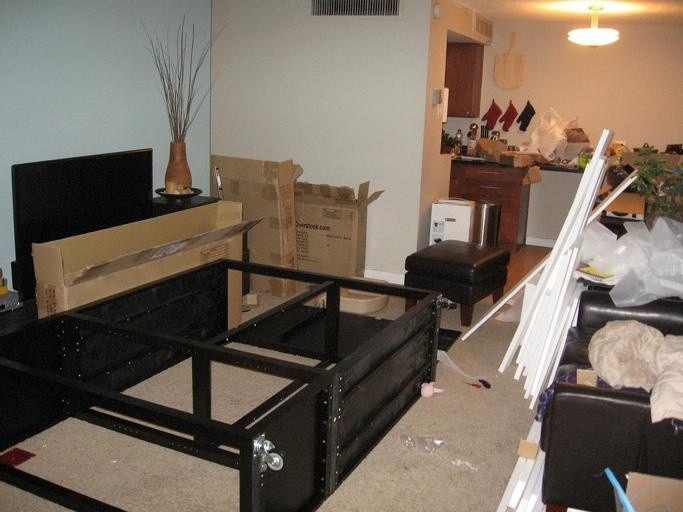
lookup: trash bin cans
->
[480,203,502,250]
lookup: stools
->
[403,239,511,327]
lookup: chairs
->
[538,289,683,511]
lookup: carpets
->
[0,283,552,512]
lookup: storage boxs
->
[555,126,591,160]
[428,198,475,246]
[597,152,657,222]
[31,200,263,331]
[209,154,303,298]
[293,180,385,288]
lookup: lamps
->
[567,6,619,47]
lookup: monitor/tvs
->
[12,148,154,302]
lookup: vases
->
[164,141,193,196]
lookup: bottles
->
[453,129,464,144]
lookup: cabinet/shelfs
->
[449,158,532,252]
